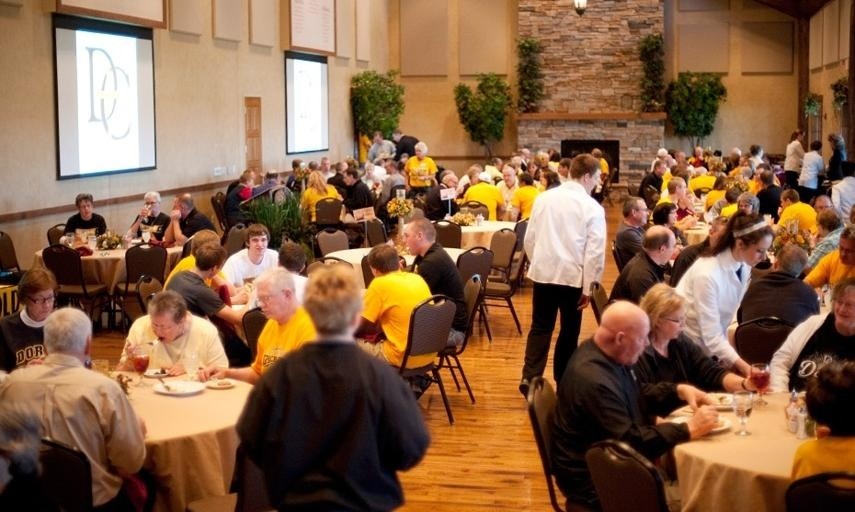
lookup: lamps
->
[574,0,587,18]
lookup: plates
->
[155,380,205,395]
[704,393,740,410]
[205,377,236,389]
[671,413,733,434]
[144,367,171,377]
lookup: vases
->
[397,217,404,237]
[459,223,469,226]
[716,172,720,178]
[300,178,306,193]
[101,244,115,249]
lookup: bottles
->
[797,408,808,440]
[784,388,800,433]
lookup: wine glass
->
[65,232,74,248]
[142,229,150,250]
[244,271,255,292]
[133,353,151,386]
[142,202,152,222]
[749,363,770,408]
[88,236,96,255]
[732,390,757,436]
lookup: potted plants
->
[637,30,667,113]
[513,36,547,114]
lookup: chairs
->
[589,280,613,329]
[514,217,530,251]
[222,222,247,255]
[627,182,638,197]
[379,184,406,231]
[645,167,651,176]
[315,227,350,256]
[784,470,854,512]
[315,197,343,226]
[485,227,518,315]
[694,187,711,198]
[317,257,354,267]
[361,253,406,289]
[39,437,93,512]
[583,439,666,511]
[438,273,485,405]
[482,245,526,335]
[216,191,225,203]
[733,317,797,367]
[611,239,623,274]
[42,243,111,331]
[431,220,460,248]
[390,294,457,426]
[455,246,495,344]
[46,223,67,246]
[145,293,155,306]
[461,199,489,221]
[526,376,581,512]
[181,236,192,259]
[0,232,28,274]
[242,306,269,361]
[112,242,168,339]
[368,188,376,203]
[211,196,229,244]
[135,274,163,315]
[643,184,658,201]
[366,217,386,246]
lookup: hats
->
[479,171,492,183]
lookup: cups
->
[91,359,110,376]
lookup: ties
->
[738,264,744,283]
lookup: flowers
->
[772,217,813,259]
[710,162,727,172]
[294,166,311,182]
[453,212,475,225]
[345,156,359,168]
[724,179,749,191]
[96,234,123,248]
[387,196,414,218]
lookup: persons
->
[706,161,727,177]
[814,195,833,213]
[649,178,688,225]
[651,147,668,173]
[850,204,855,224]
[223,174,254,231]
[440,170,454,181]
[652,202,688,247]
[220,224,280,289]
[252,169,296,204]
[459,161,491,187]
[720,187,743,216]
[405,141,438,198]
[456,167,482,199]
[519,154,606,401]
[63,194,107,237]
[163,193,218,245]
[401,217,469,350]
[0,269,57,374]
[368,131,396,166]
[674,214,775,379]
[826,133,847,181]
[302,161,324,191]
[343,168,374,221]
[804,208,847,276]
[360,162,386,198]
[1,308,146,512]
[633,283,761,416]
[116,290,230,377]
[705,177,730,211]
[798,141,825,204]
[637,161,666,209]
[777,189,819,239]
[327,161,348,189]
[424,175,460,221]
[397,153,409,175]
[285,159,305,192]
[540,172,561,190]
[756,171,783,224]
[590,148,609,203]
[197,267,321,386]
[783,129,805,189]
[690,167,717,195]
[317,156,336,184]
[226,169,256,195]
[374,160,405,216]
[770,276,855,395]
[228,265,431,512]
[751,163,780,194]
[125,191,171,242]
[735,242,820,369]
[511,174,540,221]
[510,148,561,175]
[161,229,229,292]
[791,358,855,511]
[166,244,249,324]
[724,147,741,176]
[831,161,855,226]
[737,193,760,215]
[485,157,504,186]
[669,216,730,288]
[615,196,650,268]
[609,226,676,306]
[495,166,519,209]
[803,224,855,291]
[546,302,719,512]
[727,168,755,192]
[748,144,765,173]
[686,147,709,168]
[671,152,696,179]
[728,155,752,178]
[301,171,346,225]
[352,244,432,400]
[662,165,693,194]
[251,243,308,312]
[456,172,506,221]
[558,158,571,179]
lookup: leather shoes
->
[519,379,531,399]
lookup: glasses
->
[247,237,267,243]
[26,292,57,305]
[143,201,161,207]
[663,311,689,324]
[257,292,287,306]
[150,322,179,331]
